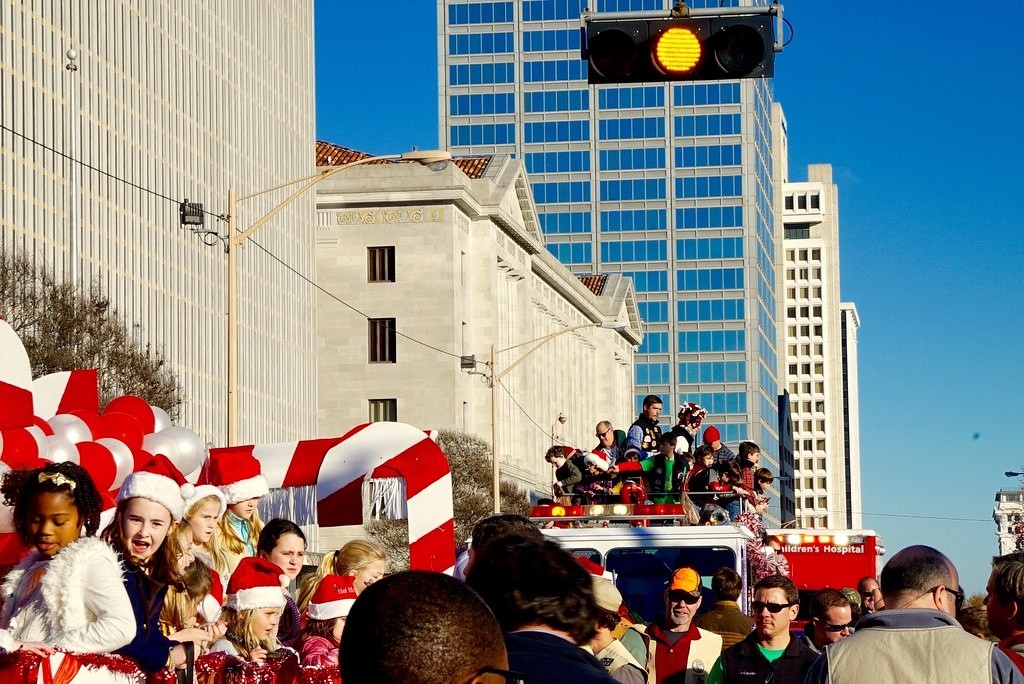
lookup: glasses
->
[861,591,872,597]
[927,585,964,610]
[467,667,526,684]
[820,621,854,631]
[667,590,699,605]
[775,550,781,554]
[751,601,796,614]
[596,428,611,438]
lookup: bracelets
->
[169,651,175,672]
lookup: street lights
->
[226,149,452,438]
[489,321,628,514]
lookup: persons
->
[468,534,622,684]
[694,567,755,650]
[803,545,1024,684]
[338,568,525,684]
[589,576,651,684]
[463,513,544,576]
[802,576,885,648]
[762,536,781,555]
[635,567,723,684]
[958,550,1024,674]
[708,574,822,684]
[545,395,774,525]
[0,451,386,672]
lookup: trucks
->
[525,504,886,633]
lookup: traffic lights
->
[584,12,775,86]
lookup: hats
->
[677,401,708,430]
[839,588,862,610]
[562,446,576,460]
[111,453,195,537]
[591,577,622,613]
[703,426,720,444]
[584,449,610,471]
[664,568,702,598]
[225,557,287,610]
[306,575,358,620]
[192,569,224,625]
[184,484,227,523]
[208,454,267,502]
[624,444,642,459]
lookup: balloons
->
[0,395,204,565]
